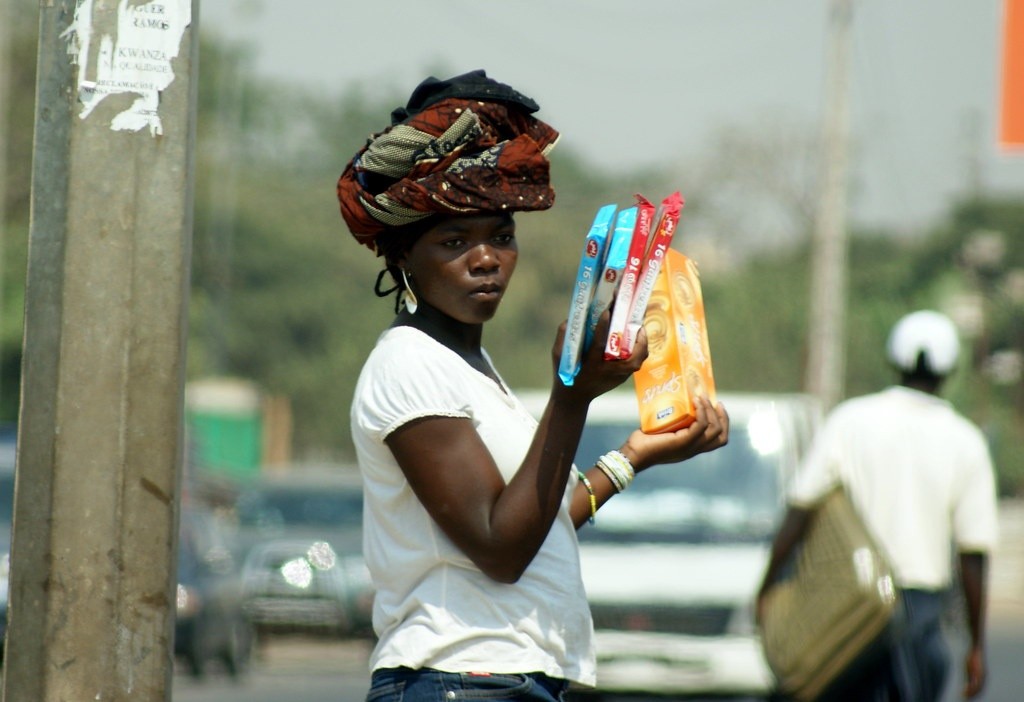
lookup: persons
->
[335,69,730,701]
[754,309,1000,702]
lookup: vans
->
[509,385,829,697]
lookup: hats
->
[886,310,962,378]
[337,69,558,257]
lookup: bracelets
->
[579,472,597,526]
[596,448,635,494]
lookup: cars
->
[1,445,263,680]
[244,466,384,645]
